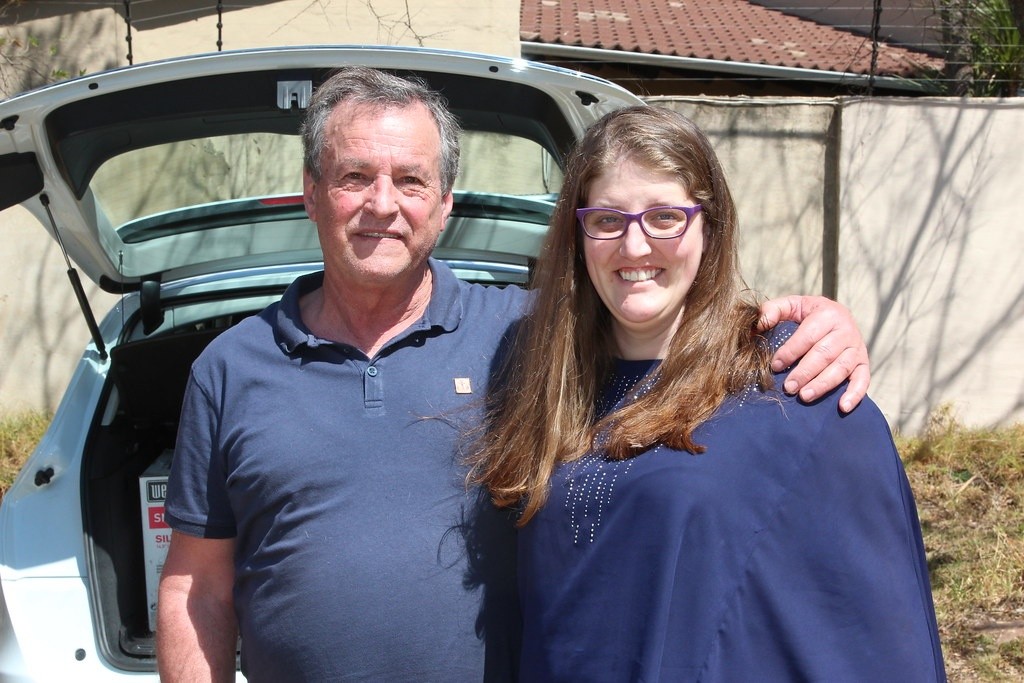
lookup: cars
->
[0,47,649,683]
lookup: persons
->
[458,106,951,682]
[156,64,871,683]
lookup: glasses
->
[575,204,704,240]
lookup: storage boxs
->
[139,446,173,633]
